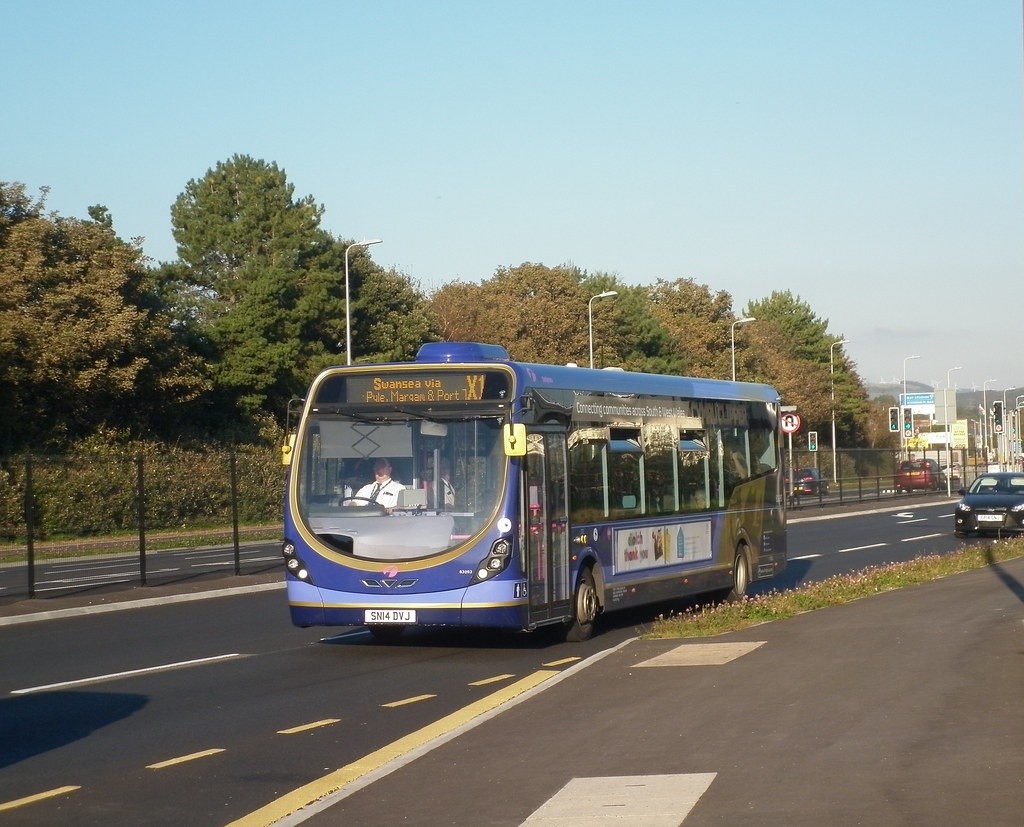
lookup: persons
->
[342,458,407,514]
[431,457,454,512]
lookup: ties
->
[448,483,451,487]
[368,483,381,507]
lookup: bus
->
[280,341,790,645]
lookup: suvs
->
[894,458,948,494]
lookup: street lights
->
[983,379,997,463]
[830,340,851,484]
[945,366,965,497]
[345,239,384,365]
[732,318,756,382]
[589,290,619,369]
[903,356,921,463]
[1003,387,1016,463]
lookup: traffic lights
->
[903,407,914,438]
[1011,427,1017,434]
[808,432,818,452]
[993,401,1004,434]
[888,407,900,432]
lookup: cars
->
[954,472,1024,539]
[942,461,965,481]
[784,466,830,500]
